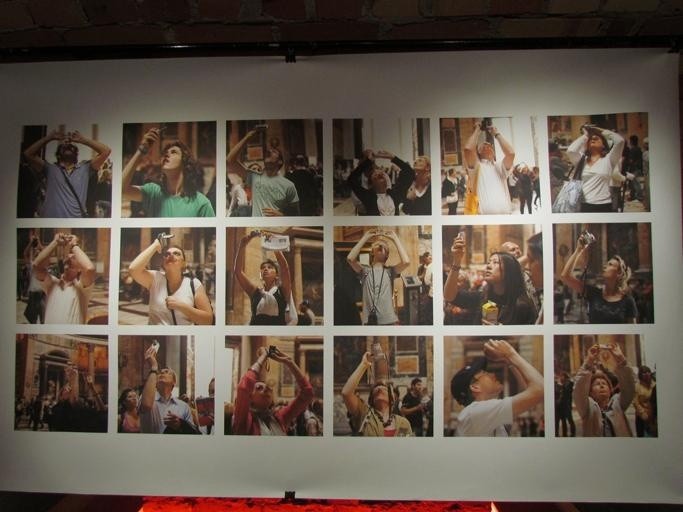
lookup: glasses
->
[257,384,273,392]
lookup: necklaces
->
[165,184,182,196]
[62,165,75,171]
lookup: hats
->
[451,356,485,404]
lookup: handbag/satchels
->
[553,180,582,212]
[464,193,480,215]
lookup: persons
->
[339,346,414,437]
[232,229,292,325]
[553,341,658,437]
[127,233,214,324]
[229,344,315,436]
[14,360,107,432]
[118,378,214,434]
[225,395,322,436]
[137,345,199,434]
[28,233,96,324]
[461,120,516,215]
[444,407,543,436]
[120,153,164,218]
[553,269,654,324]
[449,337,545,436]
[343,376,432,437]
[441,230,543,324]
[121,126,215,218]
[223,151,323,216]
[300,298,315,326]
[20,125,112,217]
[117,261,214,308]
[265,135,285,165]
[439,160,540,214]
[559,234,639,324]
[296,302,311,325]
[547,123,649,213]
[333,144,431,215]
[221,127,300,215]
[333,226,433,324]
[19,234,49,323]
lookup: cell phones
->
[457,231,465,241]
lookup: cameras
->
[151,339,160,352]
[254,123,269,131]
[368,342,386,362]
[580,122,591,134]
[250,229,262,238]
[62,132,73,142]
[584,229,597,248]
[375,227,387,236]
[268,345,277,357]
[600,343,613,349]
[480,119,492,130]
[156,232,175,248]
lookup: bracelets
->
[506,363,515,370]
[493,132,501,138]
[146,367,159,376]
[253,360,263,369]
[136,144,148,155]
[67,241,79,252]
